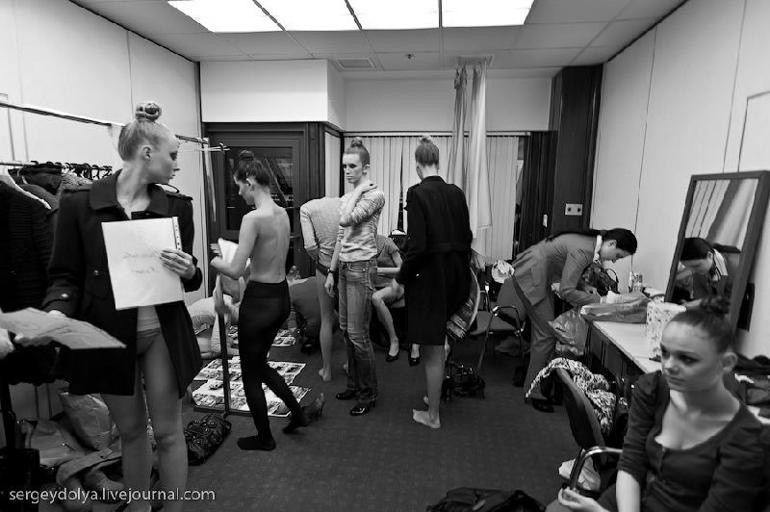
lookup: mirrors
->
[664,169,770,346]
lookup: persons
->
[324,137,386,418]
[398,132,474,431]
[13,98,203,512]
[208,147,312,450]
[543,296,770,512]
[681,237,741,318]
[299,195,533,388]
[511,226,638,413]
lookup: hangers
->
[1,159,118,205]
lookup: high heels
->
[410,354,420,366]
[386,351,400,362]
[351,401,375,415]
[524,396,554,413]
[290,393,325,421]
[336,390,359,400]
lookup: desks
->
[591,318,685,400]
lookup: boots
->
[84,459,123,503]
[56,448,123,512]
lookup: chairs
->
[470,277,525,380]
[554,363,623,502]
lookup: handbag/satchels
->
[185,412,232,466]
